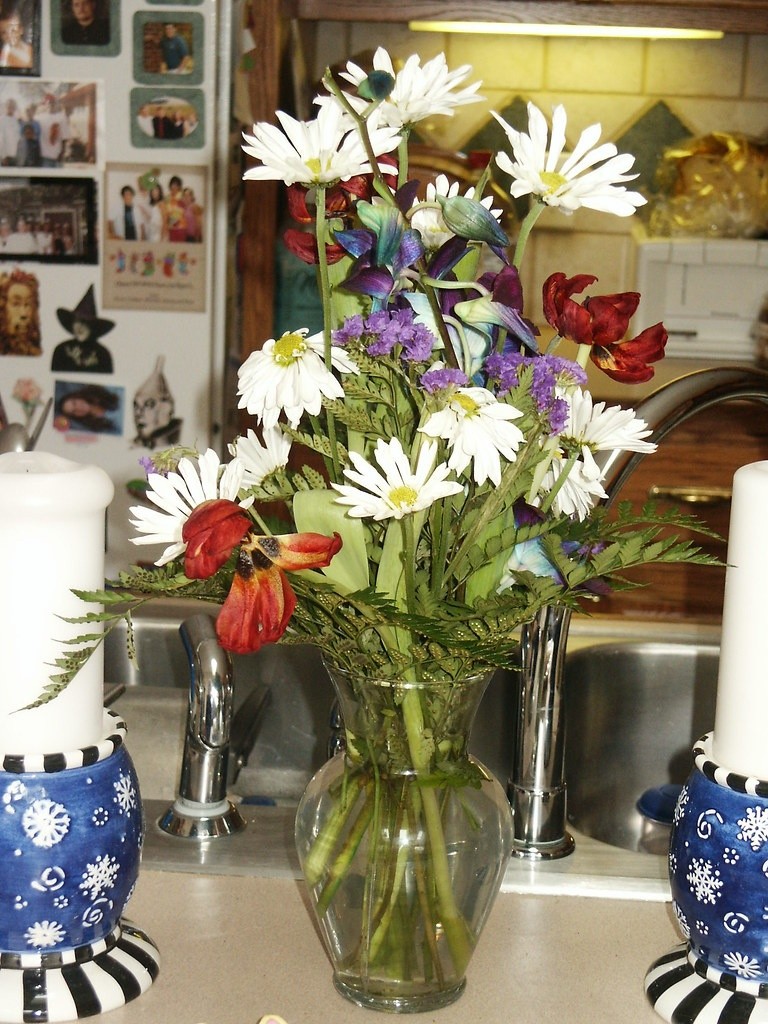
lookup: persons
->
[0,9,33,68]
[146,24,189,73]
[0,93,80,166]
[137,104,198,139]
[62,0,108,45]
[0,213,74,255]
[57,387,123,432]
[110,173,202,242]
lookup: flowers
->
[16,43,675,724]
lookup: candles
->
[712,460,768,786]
[0,449,115,753]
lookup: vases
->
[285,616,516,1013]
